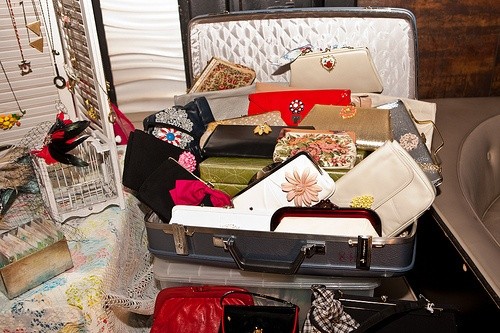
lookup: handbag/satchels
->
[310,292,461,333]
[120,43,445,235]
[218,290,300,333]
[150,284,254,333]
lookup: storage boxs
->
[153,258,380,331]
[199,149,366,200]
[0,218,74,300]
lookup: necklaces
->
[39,0,66,89]
[0,0,44,130]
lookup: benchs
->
[420,97,500,298]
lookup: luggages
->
[144,1,418,279]
